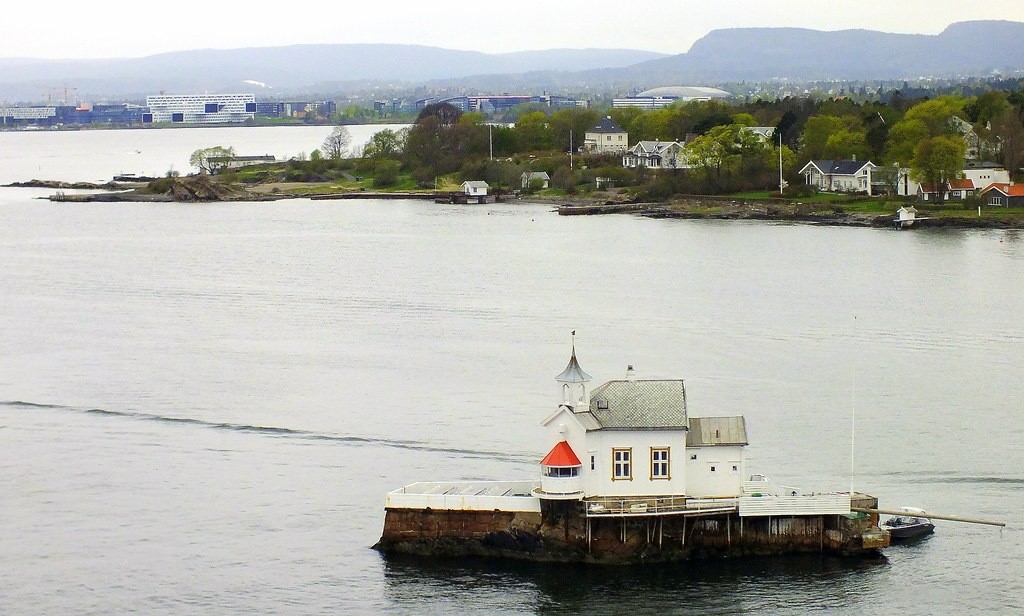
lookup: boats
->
[884,509,935,540]
[371,331,889,564]
